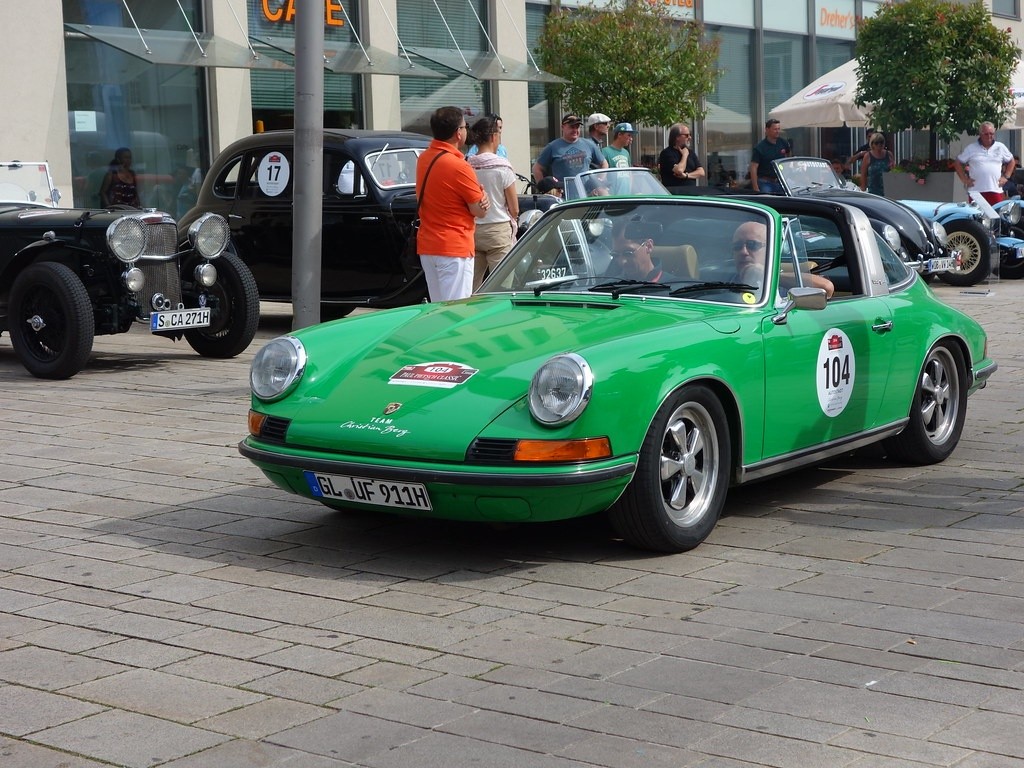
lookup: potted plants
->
[852,0,1023,203]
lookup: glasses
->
[600,122,609,125]
[680,133,691,138]
[985,132,994,136]
[733,239,765,251]
[611,242,648,259]
[456,123,469,131]
[872,141,884,146]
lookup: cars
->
[513,156,1024,287]
[1,161,260,380]
[239,194,997,557]
[176,128,563,309]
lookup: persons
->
[173,161,202,220]
[832,128,894,195]
[465,114,520,290]
[531,113,638,199]
[101,147,141,209]
[750,119,791,194]
[731,222,835,299]
[601,216,676,284]
[84,150,107,208]
[658,123,705,186]
[415,106,490,302]
[953,121,1015,206]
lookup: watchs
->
[1003,173,1010,180]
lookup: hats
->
[562,115,583,127]
[585,177,612,193]
[613,122,638,135]
[587,113,611,127]
[537,176,565,193]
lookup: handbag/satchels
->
[400,234,426,289]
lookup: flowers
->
[890,156,969,185]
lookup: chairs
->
[649,245,699,280]
[780,261,818,277]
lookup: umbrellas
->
[529,91,751,131]
[767,44,1024,130]
[400,74,486,124]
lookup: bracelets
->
[685,172,688,179]
[780,268,784,274]
